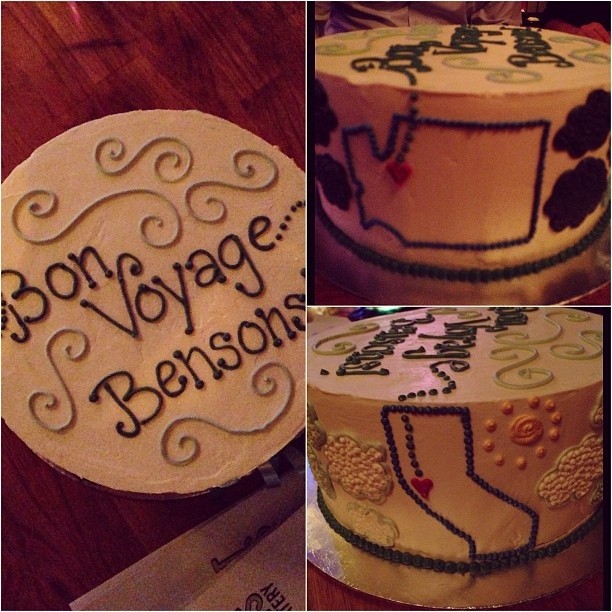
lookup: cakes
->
[0,107,305,504]
[316,22,611,306]
[308,307,604,573]
